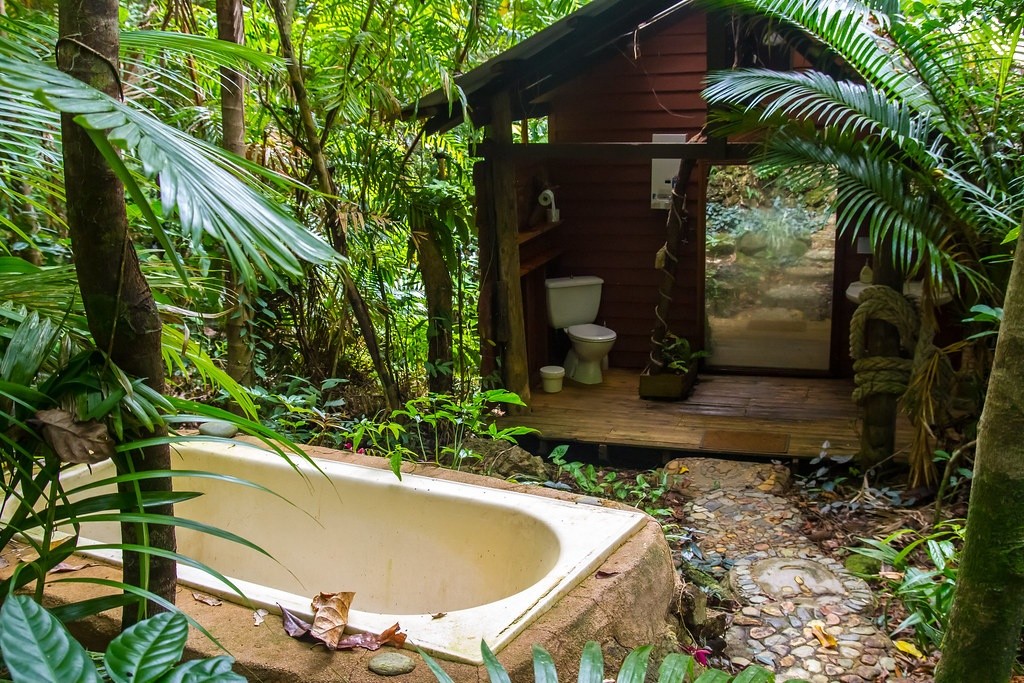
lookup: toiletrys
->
[859,254,874,284]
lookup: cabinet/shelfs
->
[513,144,579,388]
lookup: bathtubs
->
[0,410,676,683]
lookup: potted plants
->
[635,112,738,405]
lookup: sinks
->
[846,278,954,309]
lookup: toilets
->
[546,273,620,386]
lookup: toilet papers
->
[539,189,558,223]
